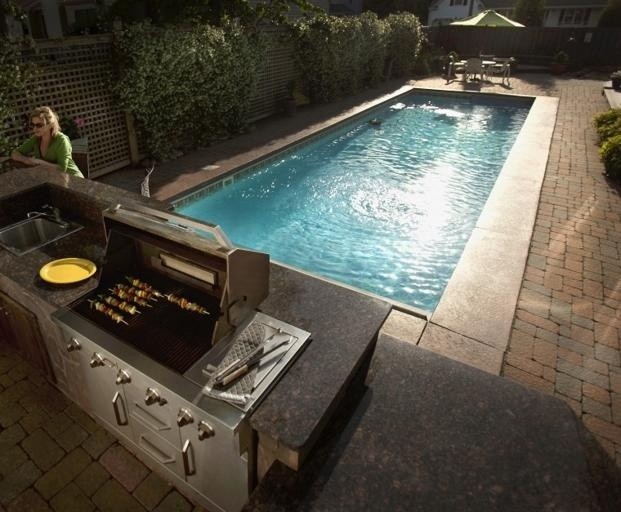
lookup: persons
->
[11,106,83,179]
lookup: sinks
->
[0,216,85,257]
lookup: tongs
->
[210,325,293,387]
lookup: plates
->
[38,256,97,285]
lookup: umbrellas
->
[449,11,527,54]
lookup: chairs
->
[444,51,517,89]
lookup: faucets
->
[26,204,61,223]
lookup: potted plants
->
[284,78,297,116]
[550,48,571,73]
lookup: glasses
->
[31,123,43,129]
